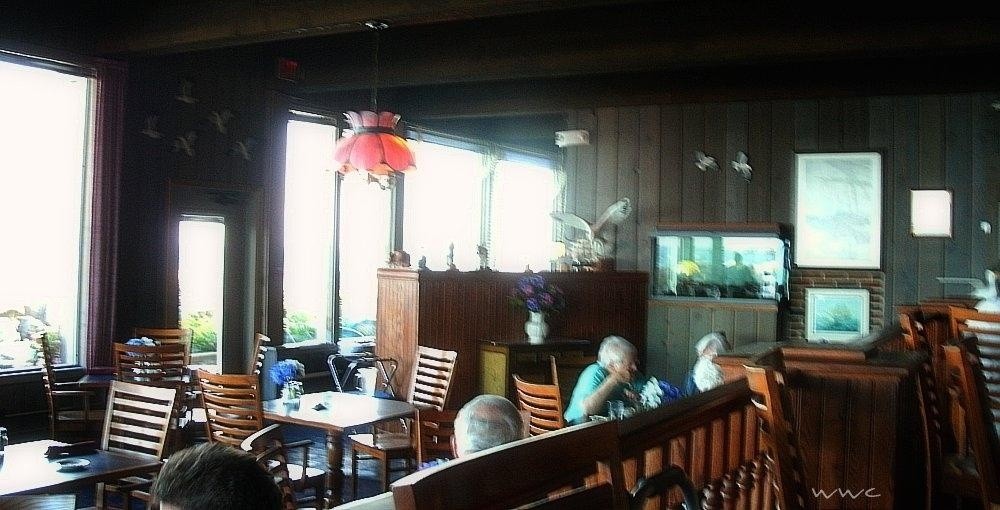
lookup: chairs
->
[513,372,566,440]
[113,342,193,460]
[131,327,191,348]
[347,338,459,500]
[238,421,345,507]
[198,369,327,510]
[190,331,272,446]
[35,330,106,442]
[72,378,176,510]
[393,419,632,509]
[728,301,999,510]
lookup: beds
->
[243,389,421,510]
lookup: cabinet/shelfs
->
[478,337,590,418]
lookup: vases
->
[280,378,303,410]
[524,295,550,343]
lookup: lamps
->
[331,17,420,191]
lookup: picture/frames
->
[804,288,871,345]
[793,154,884,271]
[911,189,952,239]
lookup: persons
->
[151,442,285,509]
[726,253,756,297]
[563,334,640,427]
[681,329,732,399]
[449,394,525,461]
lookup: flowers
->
[507,271,569,320]
[268,357,307,388]
